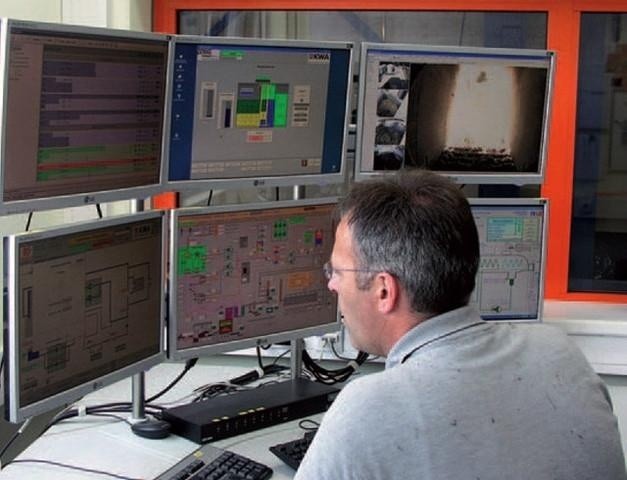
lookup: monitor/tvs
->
[0,17,173,216]
[357,40,556,185]
[167,203,344,361]
[464,194,550,329]
[172,33,354,189]
[2,211,167,425]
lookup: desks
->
[1,361,358,479]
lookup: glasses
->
[323,260,380,280]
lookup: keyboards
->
[272,437,311,471]
[155,443,273,480]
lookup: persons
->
[290,167,626,480]
